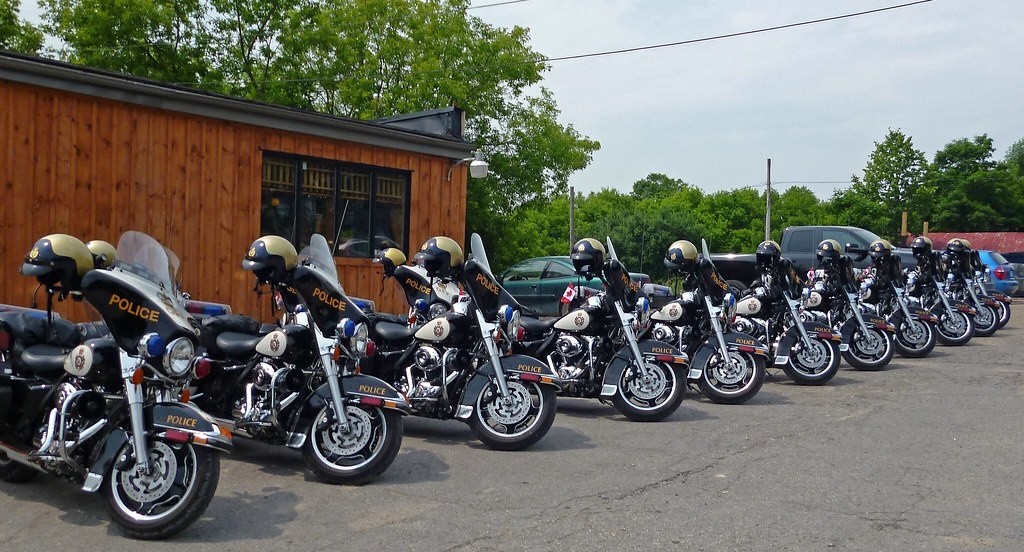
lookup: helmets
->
[910,237,932,257]
[961,239,971,250]
[869,239,891,260]
[240,235,299,288]
[372,247,406,277]
[414,236,464,284]
[664,240,698,270]
[571,238,606,273]
[947,238,964,255]
[757,241,782,266]
[86,240,116,271]
[19,234,95,302]
[815,239,842,266]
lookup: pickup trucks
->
[702,225,919,299]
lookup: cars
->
[974,249,1020,296]
[1009,262,1024,298]
[495,255,609,319]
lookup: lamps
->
[446,149,489,182]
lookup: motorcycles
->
[901,236,979,346]
[846,239,942,358]
[935,235,1014,338]
[787,239,900,372]
[0,229,234,541]
[178,233,413,486]
[719,241,844,387]
[633,238,770,406]
[342,232,563,450]
[487,236,691,423]
